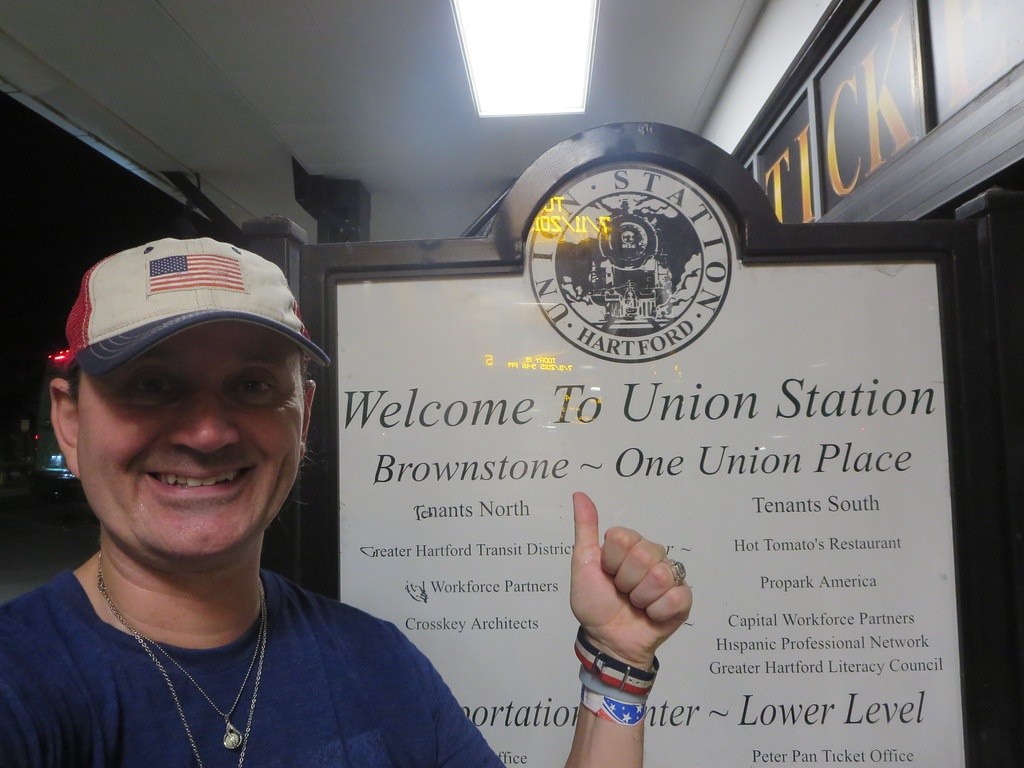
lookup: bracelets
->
[573,625,660,726]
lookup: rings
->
[663,557,687,588]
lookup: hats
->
[67,238,331,370]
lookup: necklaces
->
[98,551,268,768]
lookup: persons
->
[0,238,691,768]
